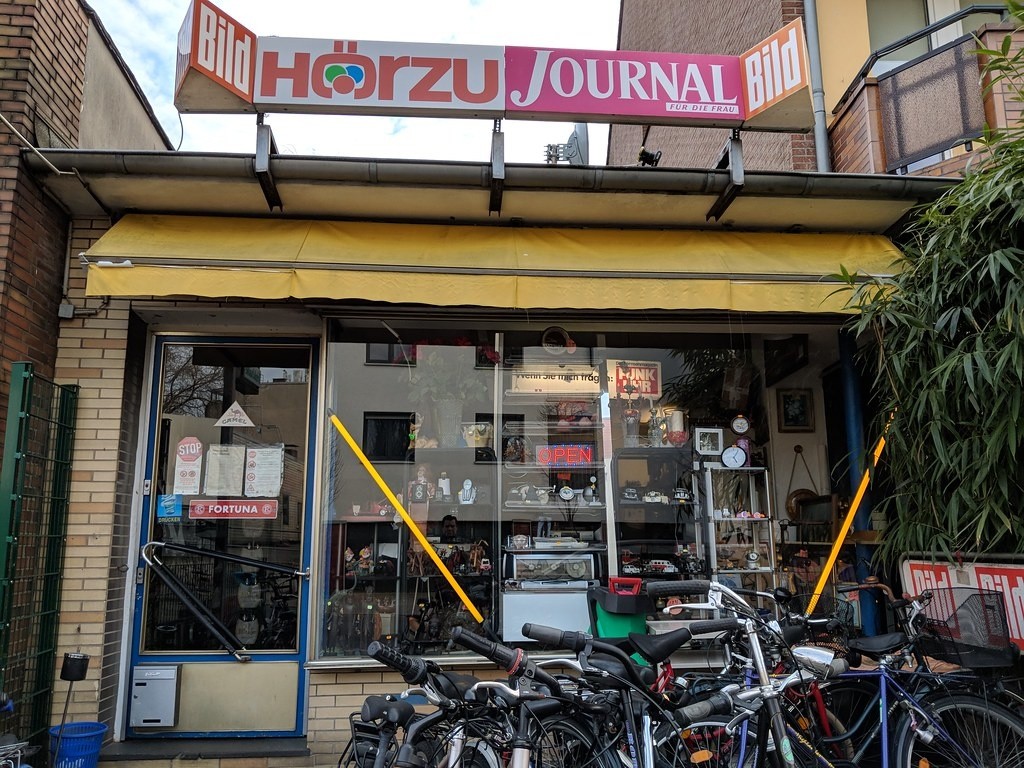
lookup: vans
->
[651,560,680,573]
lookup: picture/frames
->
[776,388,815,433]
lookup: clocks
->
[730,415,751,436]
[721,445,747,468]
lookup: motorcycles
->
[406,539,488,574]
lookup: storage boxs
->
[498,589,594,642]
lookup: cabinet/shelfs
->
[315,317,886,666]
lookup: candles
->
[672,411,683,432]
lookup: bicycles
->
[338,581,1023,768]
[216,574,492,656]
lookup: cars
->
[622,487,638,500]
[623,565,640,574]
[642,491,671,507]
[347,500,394,516]
[673,487,689,500]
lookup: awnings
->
[83,208,920,320]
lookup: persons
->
[442,513,457,543]
[402,462,435,577]
[361,585,378,650]
[327,602,343,654]
[342,588,359,649]
